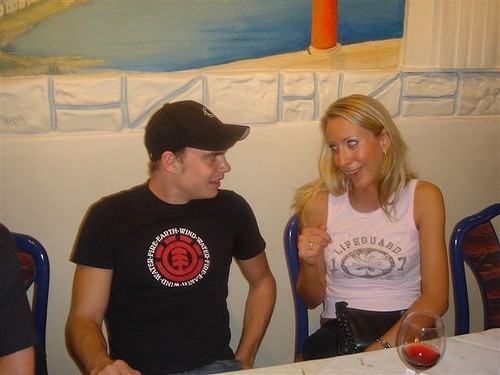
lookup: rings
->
[310,243,312,249]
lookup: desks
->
[214,327,500,375]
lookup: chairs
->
[11,232,50,347]
[449,203,500,336]
[283,214,309,362]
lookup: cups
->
[397,312,446,375]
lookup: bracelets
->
[376,338,392,349]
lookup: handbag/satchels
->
[334,300,409,356]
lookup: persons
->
[64,100,277,375]
[290,94,449,361]
[0,224,38,375]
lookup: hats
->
[144,100,250,160]
